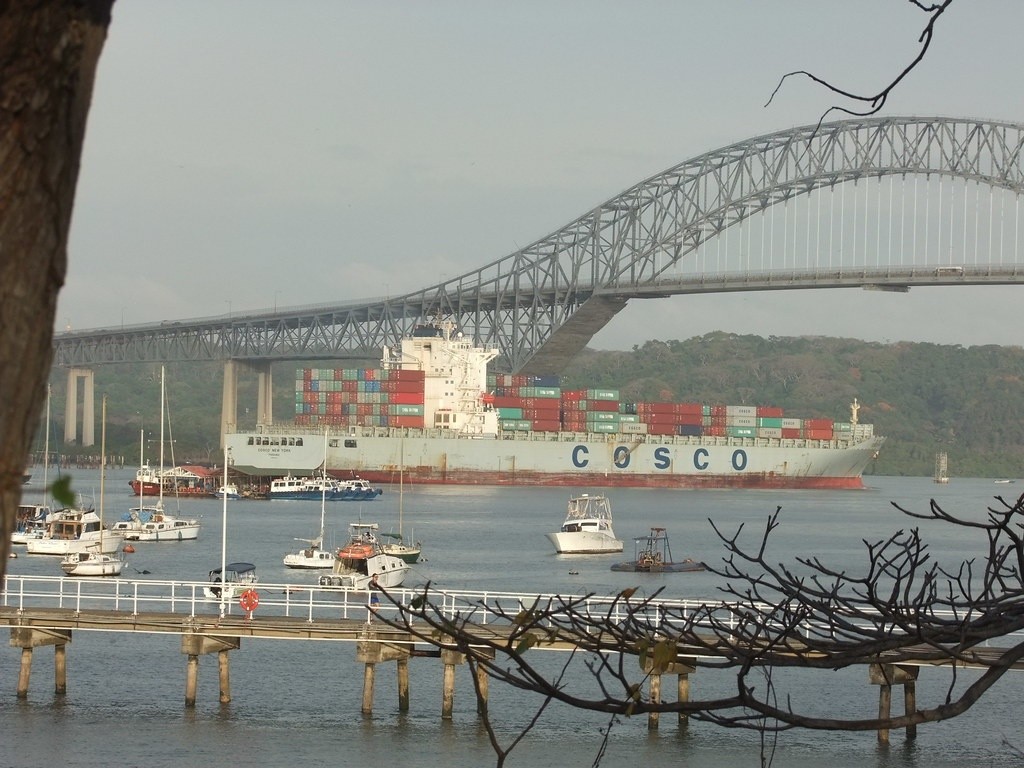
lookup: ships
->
[225,313,889,491]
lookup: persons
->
[367,573,380,623]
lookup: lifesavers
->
[241,591,259,611]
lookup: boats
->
[993,478,1016,483]
[542,492,624,553]
[608,526,708,572]
[9,363,421,607]
